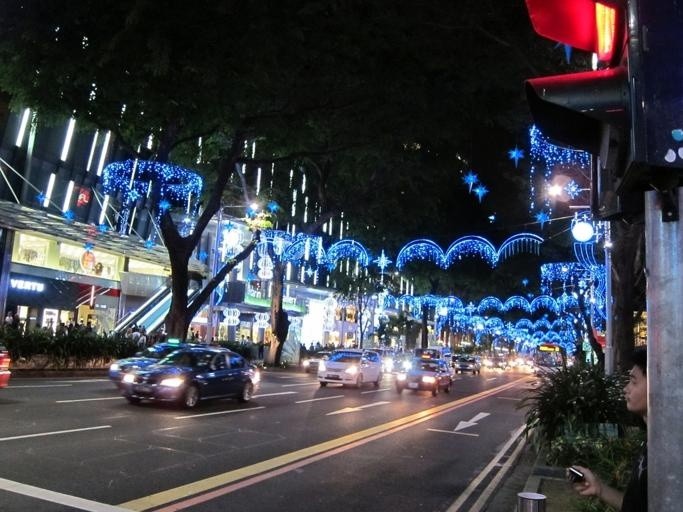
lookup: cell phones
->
[569,467,584,482]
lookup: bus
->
[532,342,567,378]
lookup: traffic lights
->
[523,1,682,191]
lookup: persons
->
[5,311,24,334]
[56,320,93,338]
[564,349,648,510]
[299,342,358,363]
[240,335,271,360]
[125,323,146,349]
[191,331,199,342]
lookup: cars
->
[297,346,539,396]
[0,338,13,391]
[565,358,574,370]
[115,344,263,408]
[103,337,233,386]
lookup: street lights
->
[205,202,259,341]
[562,199,618,376]
